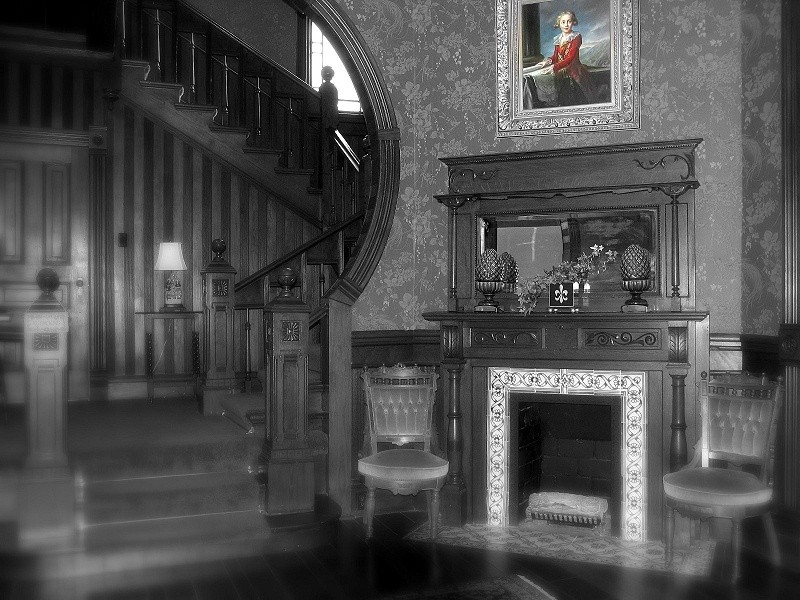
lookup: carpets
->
[402,520,717,580]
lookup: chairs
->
[662,369,786,600]
[357,360,449,541]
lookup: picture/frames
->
[494,0,643,140]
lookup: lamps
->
[154,242,189,313]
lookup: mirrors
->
[477,205,664,297]
